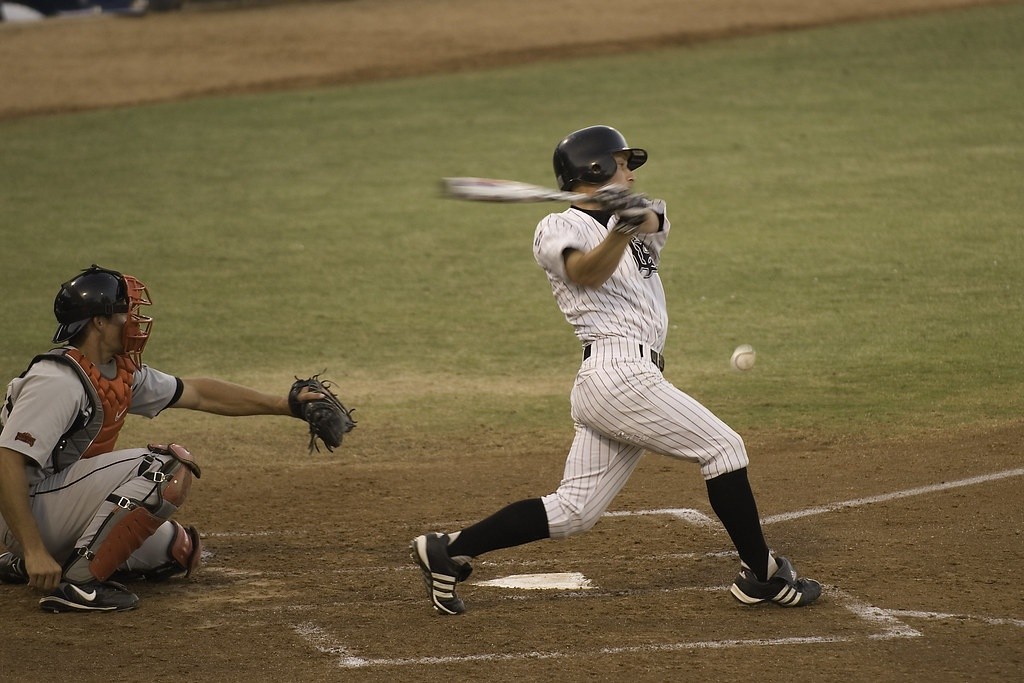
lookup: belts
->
[584,343,664,372]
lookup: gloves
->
[596,182,649,233]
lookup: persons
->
[410,125,822,622]
[0,263,327,612]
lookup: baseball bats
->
[437,175,596,206]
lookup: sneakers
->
[410,532,472,616]
[731,556,822,607]
[38,582,138,611]
[0,553,29,580]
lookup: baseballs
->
[730,344,757,372]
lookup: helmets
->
[553,124,648,191]
[50,269,128,344]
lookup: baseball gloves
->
[287,367,360,455]
